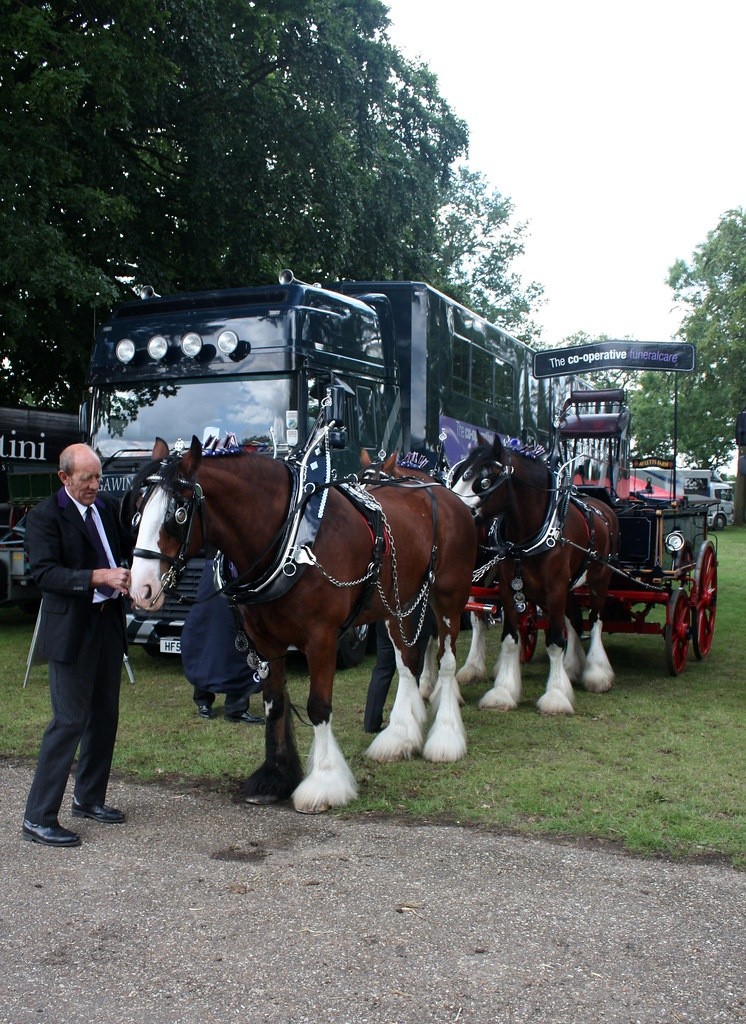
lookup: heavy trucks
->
[81,271,634,665]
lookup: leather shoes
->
[227,710,264,724]
[72,796,126,823]
[22,819,82,847]
[198,705,213,717]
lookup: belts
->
[88,602,120,613]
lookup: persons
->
[179,558,265,726]
[21,441,132,848]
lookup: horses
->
[120,426,621,816]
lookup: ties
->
[84,507,116,597]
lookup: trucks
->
[629,469,735,532]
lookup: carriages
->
[118,340,722,816]
